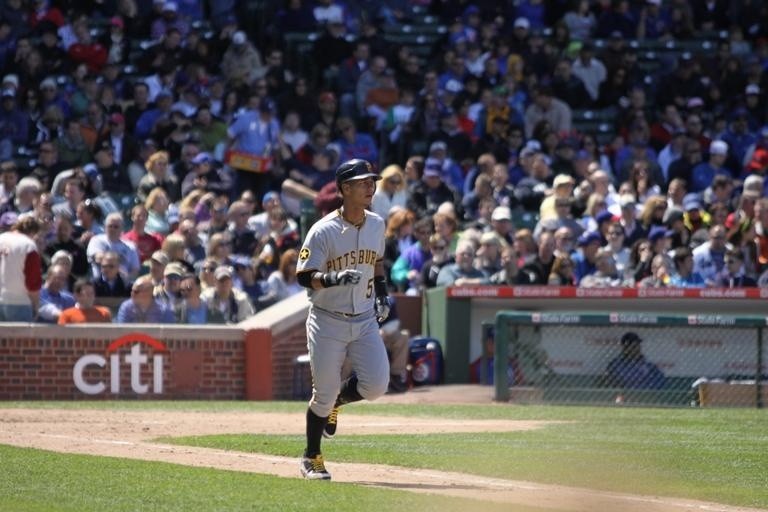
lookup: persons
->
[606,332,668,388]
[3,0,768,352]
[374,293,411,394]
[293,158,392,480]
[471,325,526,388]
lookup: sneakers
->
[301,451,331,482]
[324,405,338,438]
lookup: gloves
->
[375,295,390,322]
[322,269,362,288]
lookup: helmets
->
[335,159,382,184]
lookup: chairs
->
[0,2,767,320]
